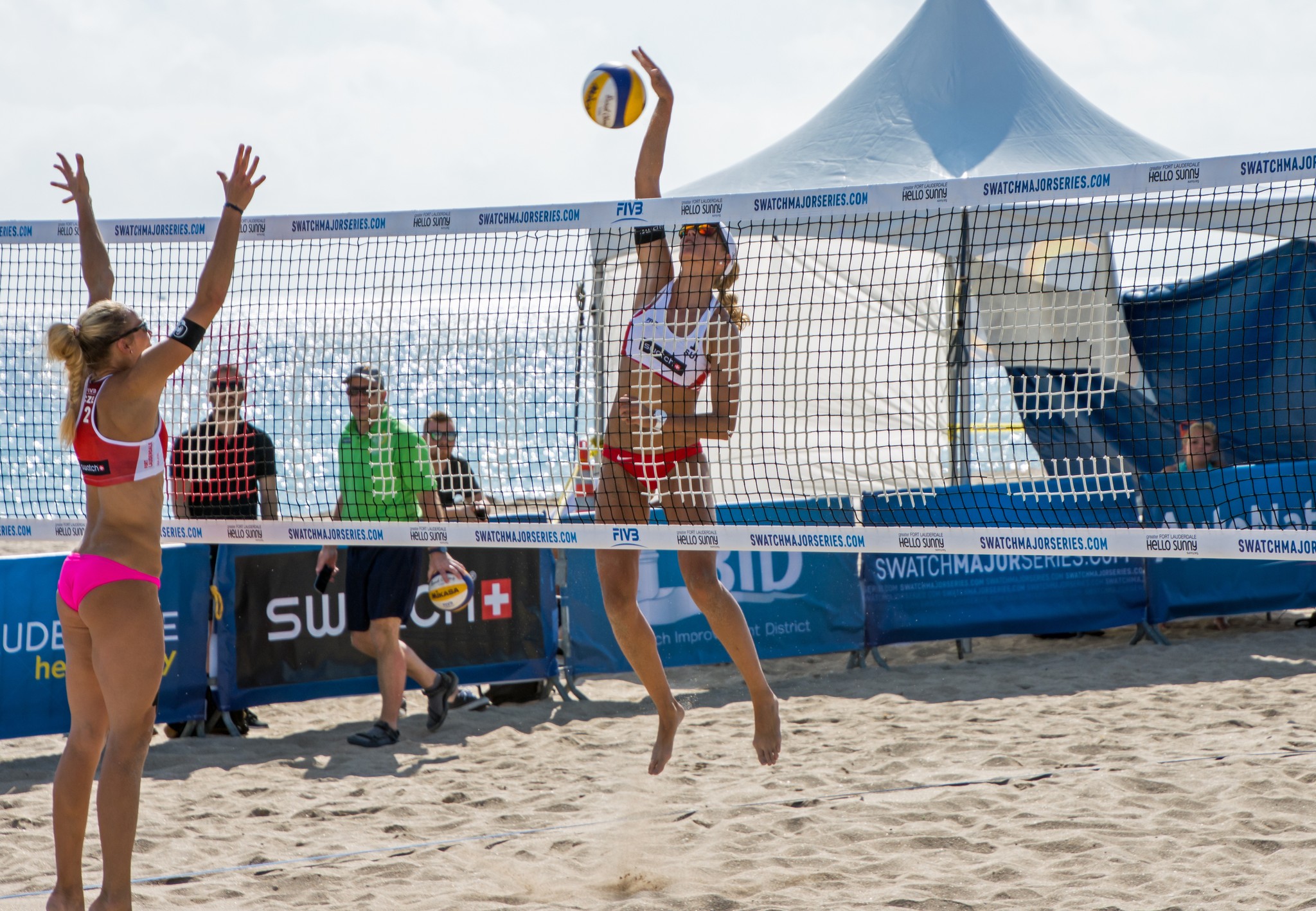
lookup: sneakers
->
[446,688,490,714]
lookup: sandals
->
[347,720,401,747]
[421,670,459,731]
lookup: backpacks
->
[163,688,249,738]
[477,679,547,705]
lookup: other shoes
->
[398,697,408,718]
[245,709,269,728]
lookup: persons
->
[45,144,266,910]
[1160,419,1225,469]
[597,48,780,775]
[171,364,493,752]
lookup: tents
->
[578,0,1316,638]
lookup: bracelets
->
[223,203,244,215]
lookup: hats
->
[342,365,385,389]
[711,221,738,276]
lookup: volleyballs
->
[583,61,647,129]
[429,569,474,612]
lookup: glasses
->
[108,318,148,347]
[345,385,378,396]
[209,380,243,393]
[429,430,457,442]
[678,224,718,237]
